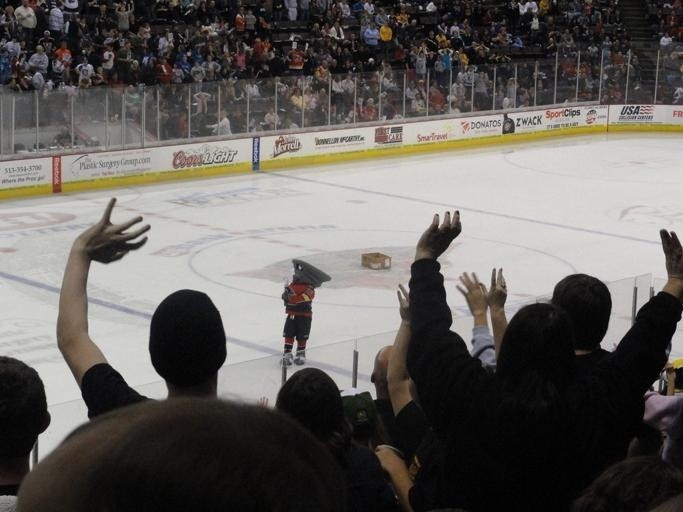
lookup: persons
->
[56,195,229,421]
[369,207,683,512]
[0,3,683,162]
[15,397,350,512]
[275,365,408,512]
[0,350,52,512]
[278,258,316,367]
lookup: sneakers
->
[295,353,306,364]
[280,354,293,365]
